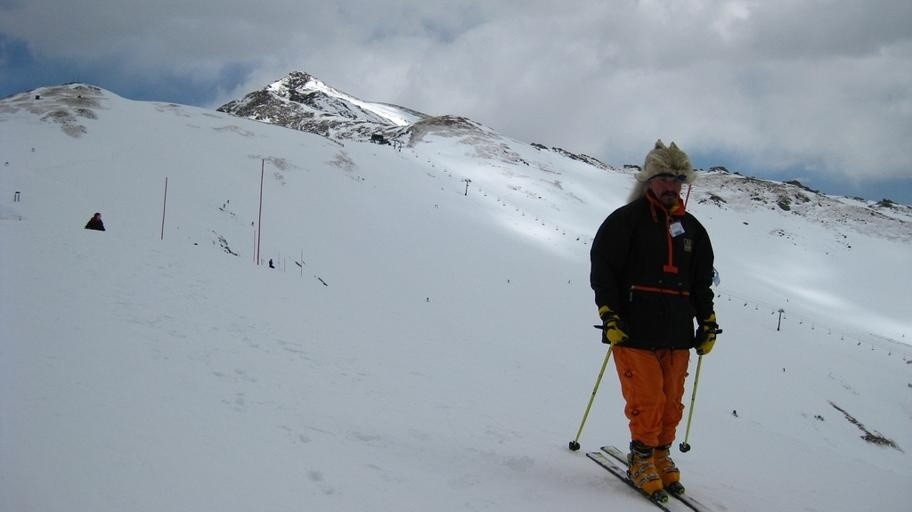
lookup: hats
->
[634,140,694,184]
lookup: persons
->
[85,212,106,231]
[589,138,723,497]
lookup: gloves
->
[695,312,717,354]
[598,304,628,347]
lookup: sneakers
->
[626,439,664,496]
[654,443,679,487]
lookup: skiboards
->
[586,447,710,512]
